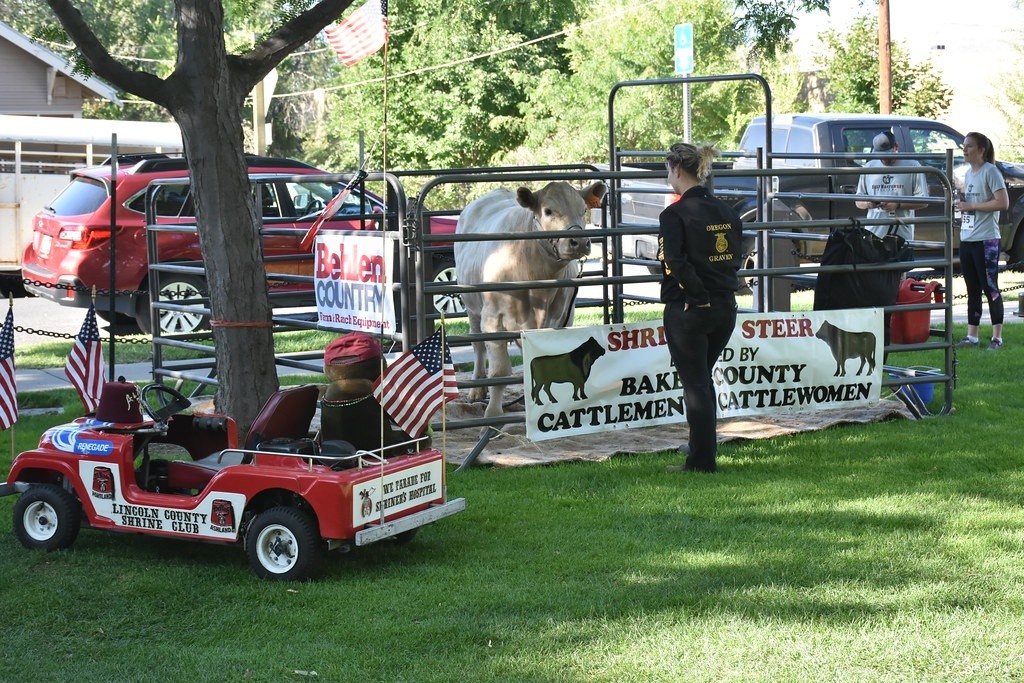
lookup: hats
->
[872,132,896,152]
[324,332,382,367]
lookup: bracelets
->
[897,203,900,209]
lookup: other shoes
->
[679,444,691,456]
[666,465,685,472]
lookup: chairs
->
[253,184,279,217]
[170,384,321,492]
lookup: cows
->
[453,182,607,439]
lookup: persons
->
[956,133,1009,351]
[855,132,930,280]
[657,144,743,473]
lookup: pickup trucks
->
[587,112,1024,296]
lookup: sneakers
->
[955,336,979,349]
[986,338,1001,352]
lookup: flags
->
[301,169,368,251]
[372,324,459,439]
[0,306,19,431]
[64,298,109,413]
[326,0,388,66]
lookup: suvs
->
[21,152,467,338]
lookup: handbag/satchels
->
[813,217,915,366]
